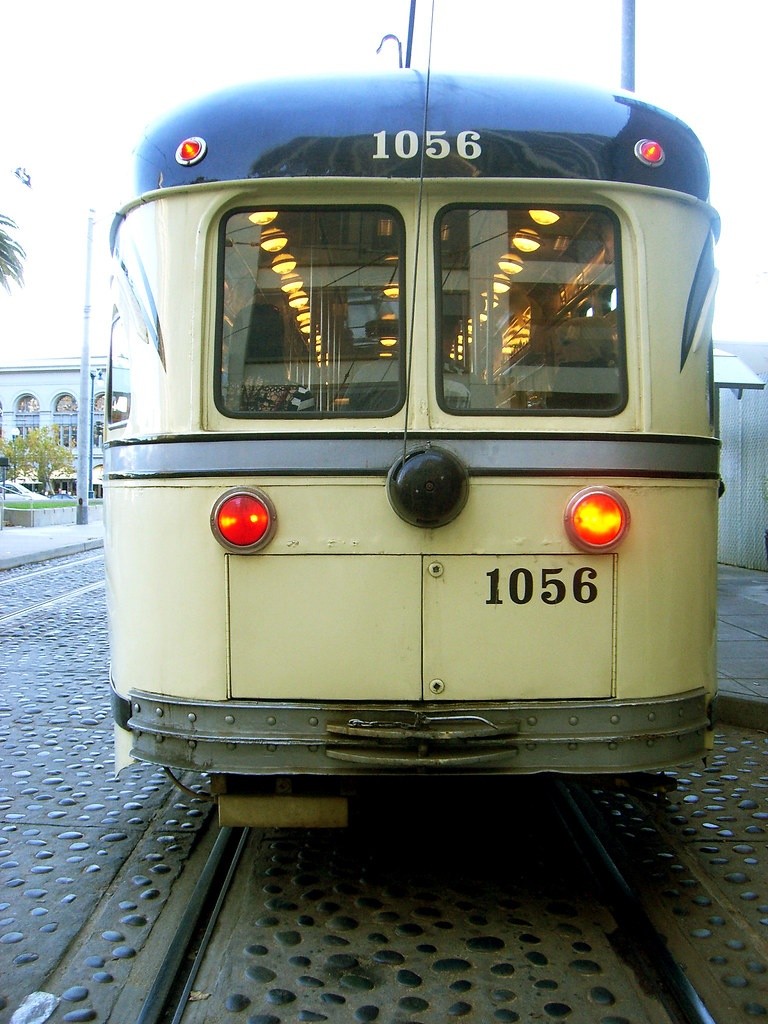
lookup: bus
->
[103,69,721,828]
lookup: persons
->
[223,302,315,412]
[546,315,617,410]
[338,300,400,413]
[442,315,472,410]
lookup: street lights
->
[88,371,104,498]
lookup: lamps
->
[249,210,561,367]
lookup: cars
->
[50,494,75,500]
[0,482,50,500]
